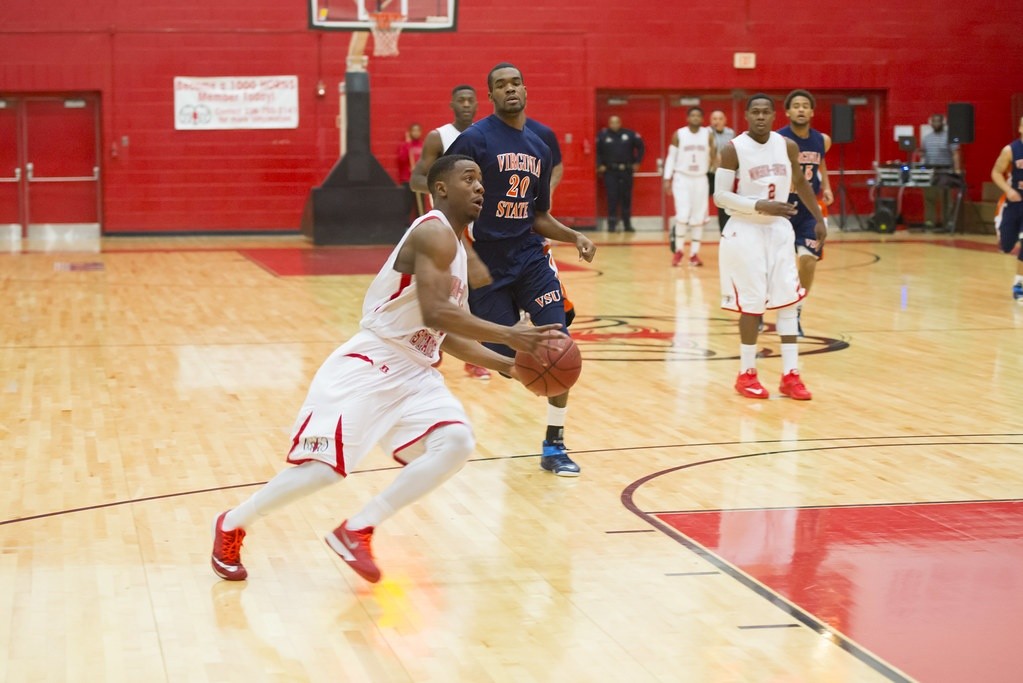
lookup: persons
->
[713,93,826,400]
[920,113,961,227]
[211,154,567,583]
[443,63,596,477]
[596,115,644,232]
[668,110,736,253]
[991,117,1023,301]
[662,106,717,267]
[398,123,425,228]
[758,89,834,337]
[410,84,492,378]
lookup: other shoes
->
[925,220,935,226]
[625,226,634,231]
[757,317,764,331]
[670,232,676,253]
[798,323,803,335]
[608,225,614,232]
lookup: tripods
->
[945,144,991,235]
[829,142,867,231]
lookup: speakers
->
[948,102,975,145]
[874,197,896,234]
[831,104,854,143]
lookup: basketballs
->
[515,329,582,398]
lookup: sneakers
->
[690,254,703,265]
[464,363,490,380]
[734,368,769,398]
[778,369,812,399]
[1013,284,1023,301]
[673,252,682,265]
[540,440,581,476]
[325,520,381,583]
[209,509,247,581]
